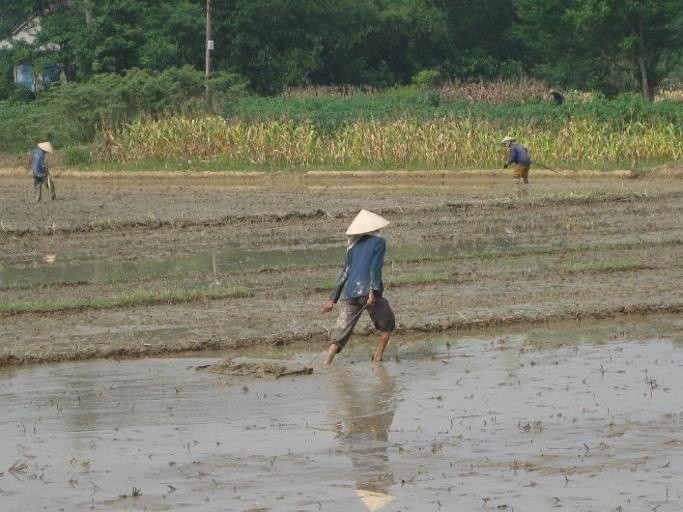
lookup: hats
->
[345,208,389,236]
[37,141,53,154]
[500,136,516,146]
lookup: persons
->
[320,209,394,365]
[32,140,56,200]
[547,89,566,105]
[498,135,531,185]
